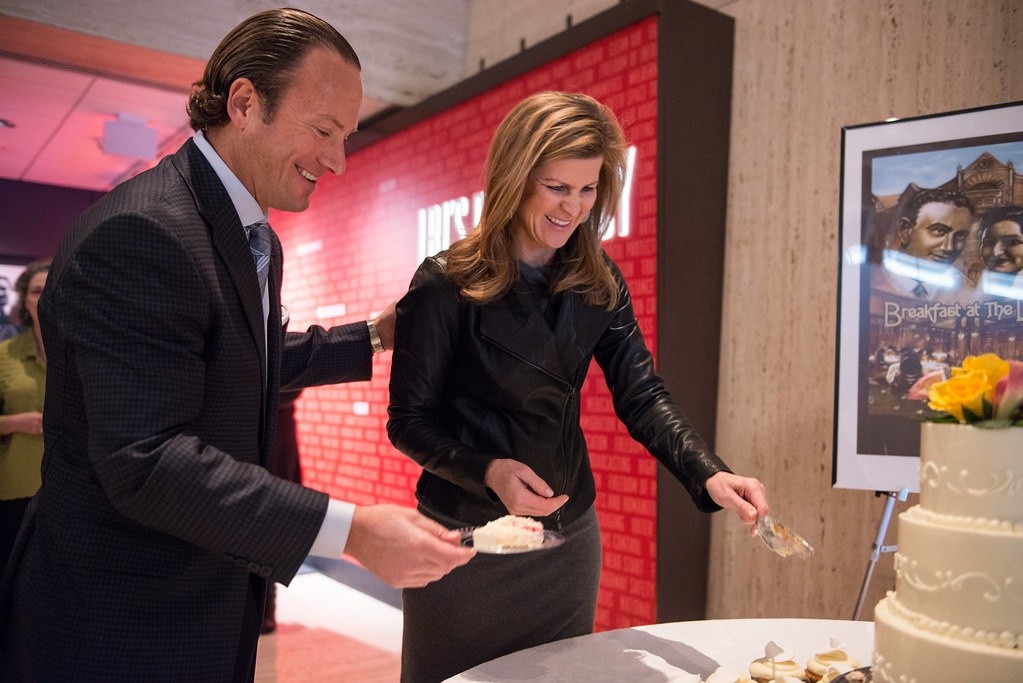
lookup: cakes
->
[473,514,545,548]
[750,422,1023,683]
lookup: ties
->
[246,222,273,301]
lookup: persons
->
[385,92,768,683]
[0,258,54,565]
[0,7,478,683]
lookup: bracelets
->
[366,319,385,355]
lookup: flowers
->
[907,353,1023,430]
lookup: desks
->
[441,617,875,683]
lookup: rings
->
[40,428,43,433]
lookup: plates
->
[453,525,565,555]
